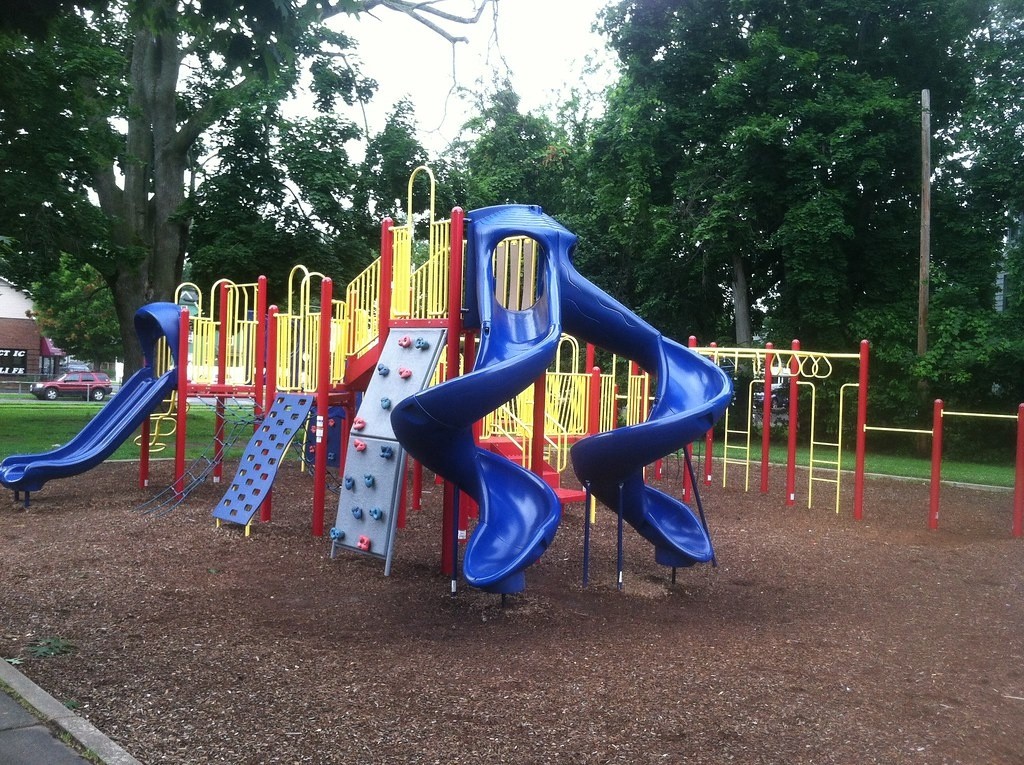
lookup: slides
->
[0,367,177,490]
[390,260,735,597]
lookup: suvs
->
[29,371,114,401]
